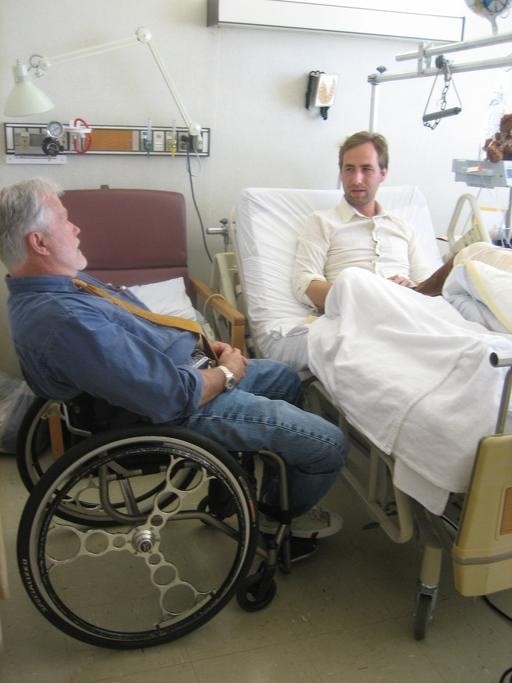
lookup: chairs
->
[49,185,247,460]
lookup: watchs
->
[218,365,236,391]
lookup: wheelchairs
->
[17,360,325,652]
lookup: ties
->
[72,279,219,365]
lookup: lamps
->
[305,69,340,119]
[4,28,202,137]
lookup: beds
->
[206,184,511,641]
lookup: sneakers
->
[259,506,344,537]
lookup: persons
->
[290,131,456,312]
[0,179,349,542]
